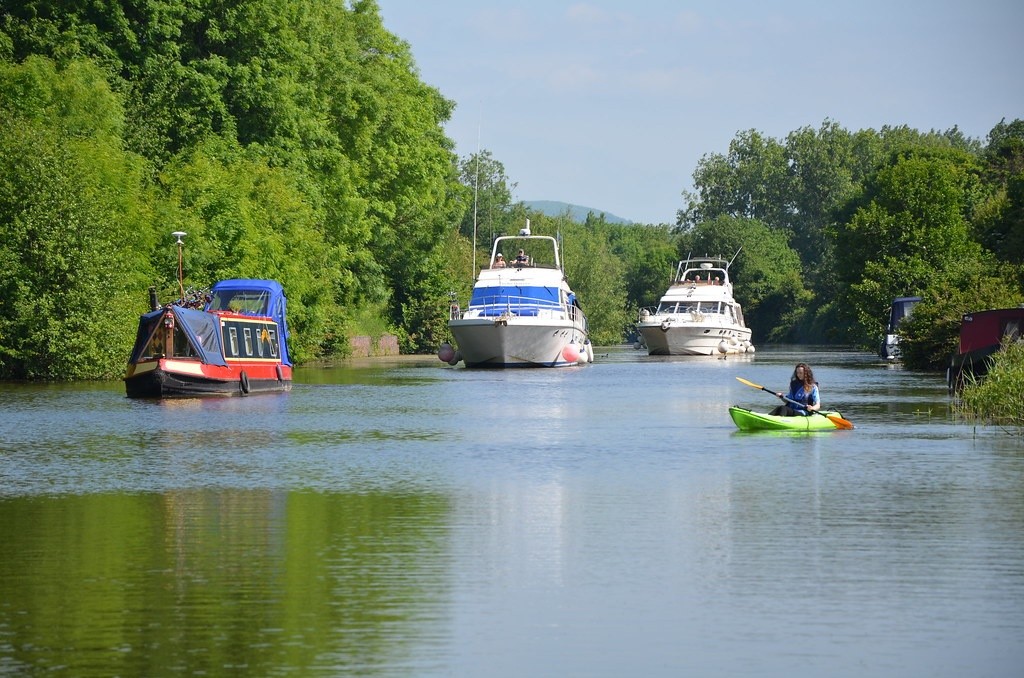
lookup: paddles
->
[735,376,853,430]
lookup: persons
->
[496,253,505,268]
[714,276,719,281]
[768,363,820,416]
[509,250,529,265]
[687,275,701,283]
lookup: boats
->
[438,128,595,370]
[728,405,844,432]
[946,307,1024,398]
[121,229,293,400]
[880,294,925,361]
[634,246,755,356]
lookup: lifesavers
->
[240,371,251,393]
